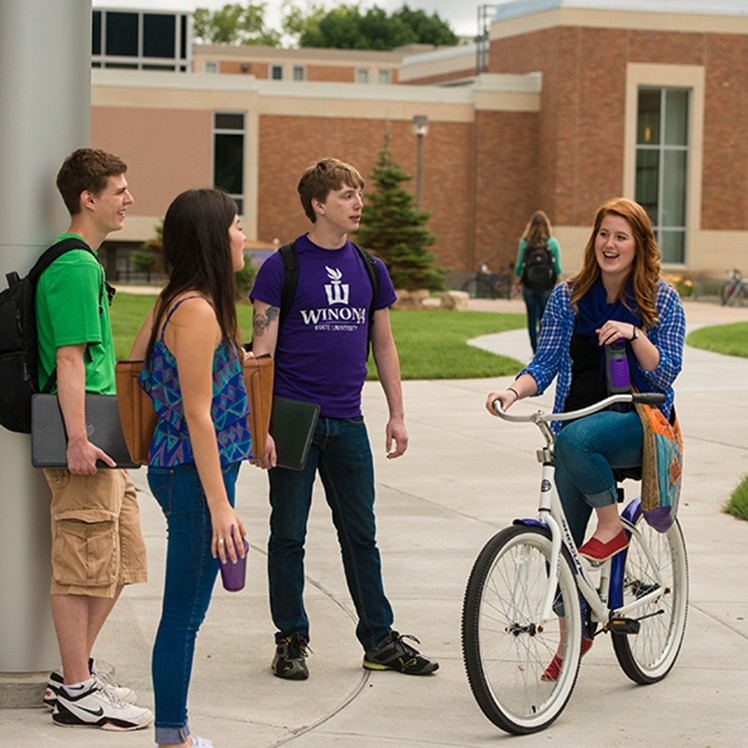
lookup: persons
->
[249,157,440,678]
[34,147,156,733]
[508,211,563,357]
[125,189,256,748]
[485,197,685,683]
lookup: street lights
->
[412,115,430,210]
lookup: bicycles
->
[720,269,748,307]
[461,392,690,735]
[462,262,514,300]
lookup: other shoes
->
[155,735,213,748]
[578,528,630,562]
[541,637,593,682]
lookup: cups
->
[217,539,249,592]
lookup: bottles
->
[604,337,635,413]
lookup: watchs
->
[629,324,639,341]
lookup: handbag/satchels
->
[114,353,275,465]
[629,376,683,534]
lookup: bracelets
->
[507,388,520,401]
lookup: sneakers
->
[271,632,313,680]
[50,680,153,732]
[363,631,439,676]
[42,658,137,709]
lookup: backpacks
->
[0,237,103,434]
[521,237,557,291]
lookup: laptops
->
[264,394,322,473]
[30,392,141,470]
